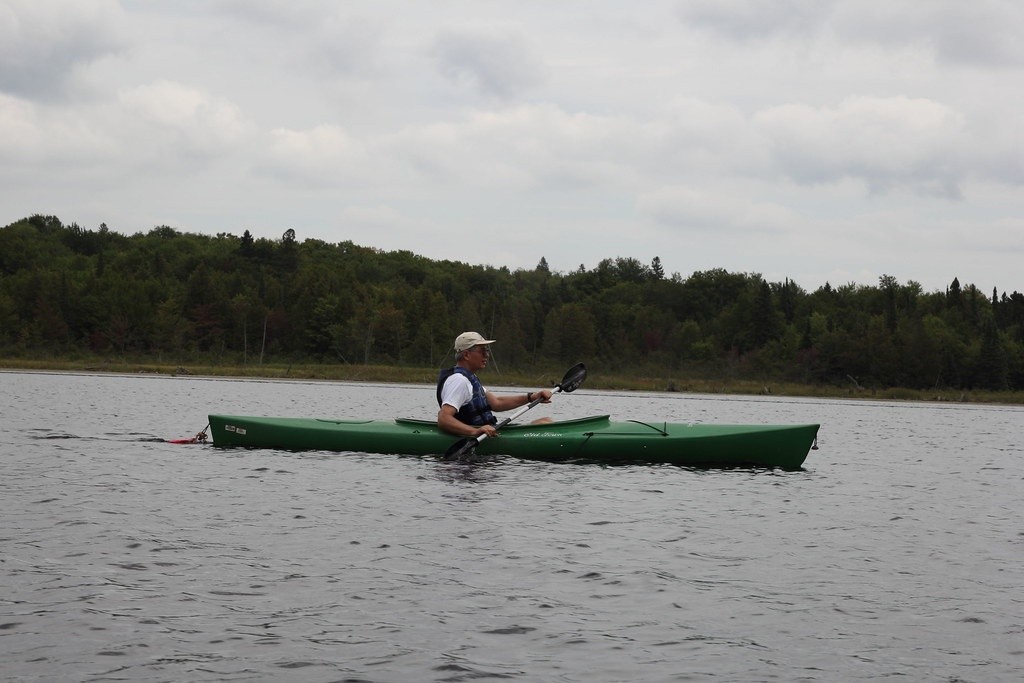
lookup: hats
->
[454,332,496,353]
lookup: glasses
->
[466,348,491,356]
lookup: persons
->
[440,331,553,440]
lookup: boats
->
[207,413,822,472]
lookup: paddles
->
[444,363,588,461]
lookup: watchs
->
[527,392,533,403]
